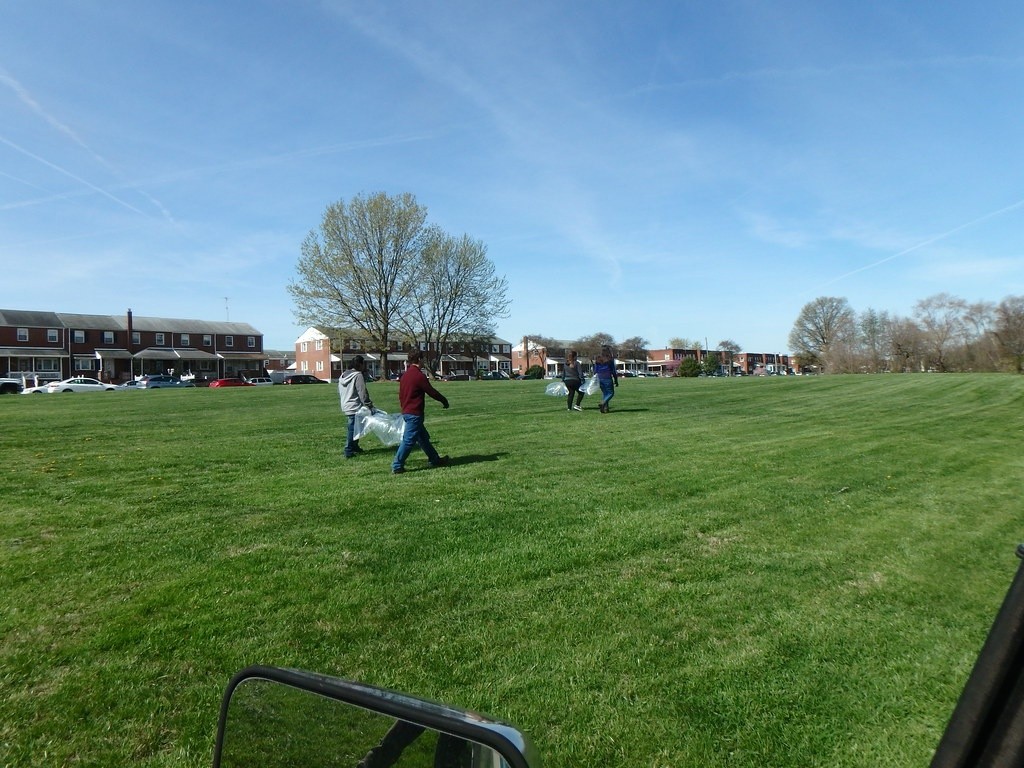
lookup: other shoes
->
[598,403,604,412]
[603,406,609,413]
[574,405,582,411]
[354,447,363,452]
[433,455,449,466]
[392,468,405,474]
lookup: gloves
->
[614,381,618,387]
[370,406,377,415]
[442,401,449,409]
[581,379,585,384]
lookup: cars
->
[282,375,328,385]
[0,378,24,395]
[136,375,196,389]
[187,379,210,387]
[48,378,121,393]
[246,378,273,386]
[21,381,61,394]
[209,378,257,387]
[516,375,529,380]
[121,380,139,390]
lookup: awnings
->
[95,351,133,359]
[0,346,69,357]
[134,348,221,360]
[546,357,644,366]
[440,354,511,362]
[331,352,409,362]
[217,353,269,361]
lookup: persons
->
[561,351,585,412]
[391,349,449,474]
[593,345,618,413]
[338,355,377,459]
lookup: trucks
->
[441,375,469,381]
[479,371,509,380]
